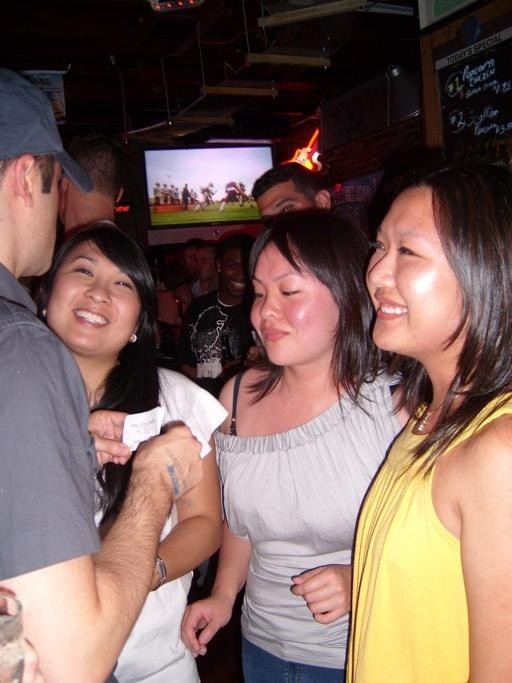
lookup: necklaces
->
[417,385,469,431]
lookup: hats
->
[0,67,94,194]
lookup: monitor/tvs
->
[132,143,278,245]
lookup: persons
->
[150,174,259,214]
[157,224,263,399]
[0,64,206,682]
[55,134,127,245]
[251,161,332,215]
[37,217,232,682]
[175,206,415,683]
[343,153,511,683]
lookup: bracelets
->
[154,552,168,590]
